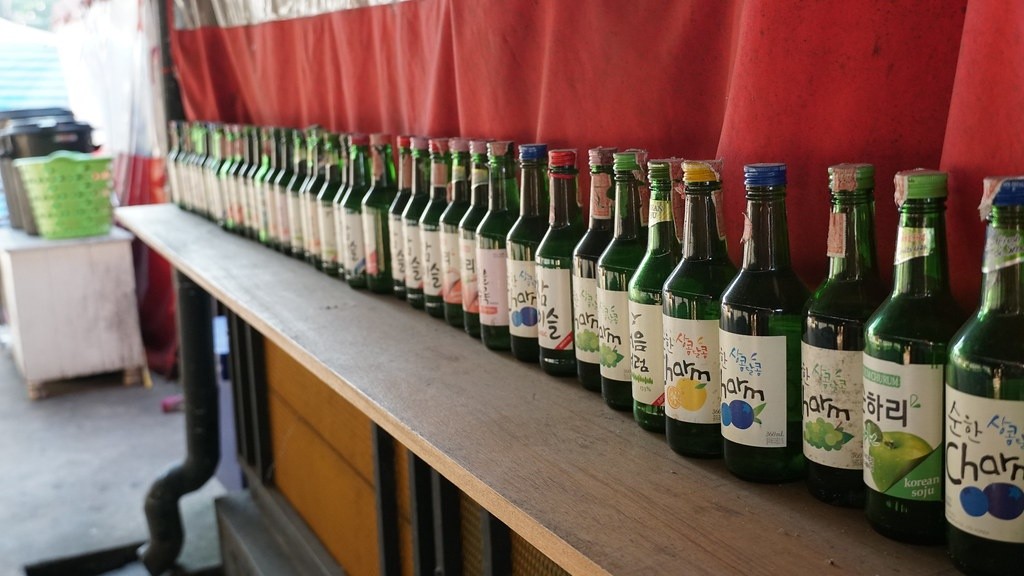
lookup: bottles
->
[165,117,1024,576]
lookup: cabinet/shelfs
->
[0,224,148,400]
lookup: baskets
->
[13,151,115,239]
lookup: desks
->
[115,202,962,576]
